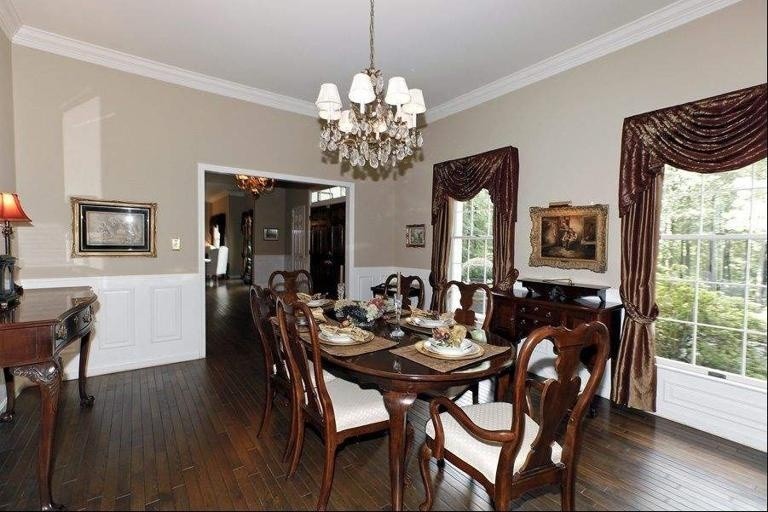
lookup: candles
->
[397,271,400,300]
[340,264,344,282]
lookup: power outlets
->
[171,239,180,250]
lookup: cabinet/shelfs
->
[1,286,97,512]
[486,288,624,419]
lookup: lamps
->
[316,0,426,169]
[233,173,275,198]
[0,192,33,256]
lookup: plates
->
[307,299,330,307]
[428,337,472,353]
[415,340,485,360]
[318,332,369,343]
[405,317,456,328]
[318,330,375,345]
[423,340,481,357]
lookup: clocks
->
[0,255,20,314]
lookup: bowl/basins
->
[319,323,339,337]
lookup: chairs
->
[268,269,313,298]
[207,245,228,286]
[250,285,338,464]
[276,293,415,512]
[384,274,425,309]
[418,321,610,512]
[440,281,493,331]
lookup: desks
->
[371,283,421,298]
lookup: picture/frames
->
[406,224,426,247]
[263,227,280,241]
[529,204,608,273]
[71,197,159,258]
